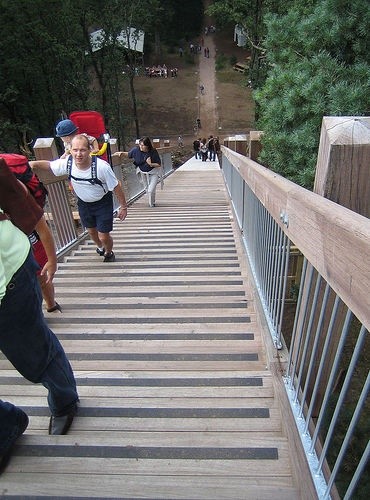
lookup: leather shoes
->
[48,403,75,435]
[0,410,29,470]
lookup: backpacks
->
[201,143,207,154]
[0,152,48,209]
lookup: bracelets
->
[120,208,127,210]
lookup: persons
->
[196,119,204,131]
[142,62,178,79]
[179,23,216,58]
[57,119,100,159]
[200,82,207,96]
[177,135,185,149]
[28,133,128,264]
[0,153,80,475]
[114,135,161,208]
[193,135,221,162]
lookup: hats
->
[55,119,79,138]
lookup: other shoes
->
[103,251,115,262]
[100,248,104,256]
[47,301,62,313]
